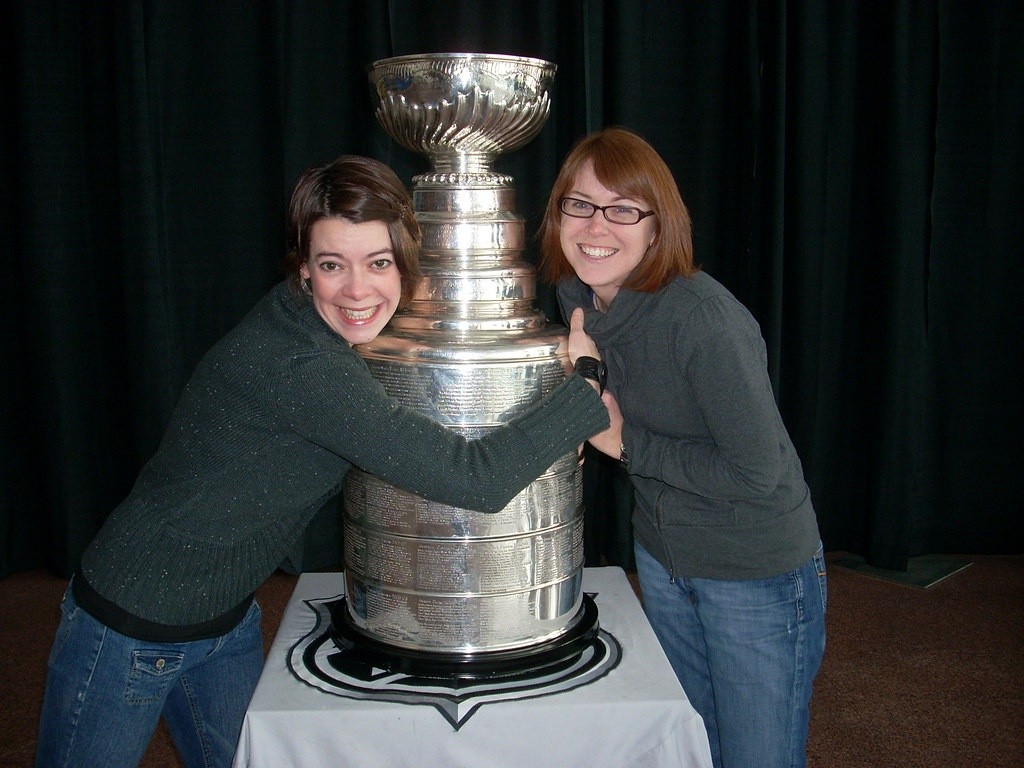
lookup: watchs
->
[575,356,608,398]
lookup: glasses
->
[557,197,656,225]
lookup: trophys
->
[329,52,600,682]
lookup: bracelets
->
[620,443,628,465]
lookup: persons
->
[538,129,826,767]
[35,156,611,768]
